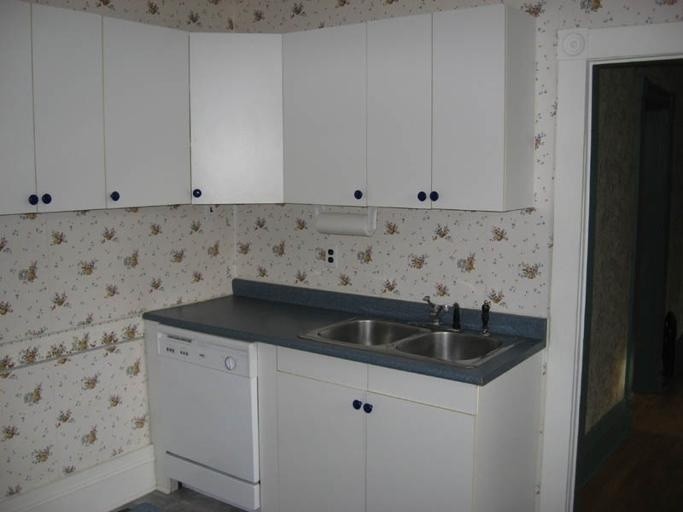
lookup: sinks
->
[396,331,523,369]
[297,314,428,346]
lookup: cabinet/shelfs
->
[103,17,191,210]
[364,4,534,213]
[256,345,542,512]
[1,0,107,214]
[190,31,284,205]
[283,22,367,207]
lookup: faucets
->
[422,295,450,325]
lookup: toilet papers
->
[316,212,373,237]
